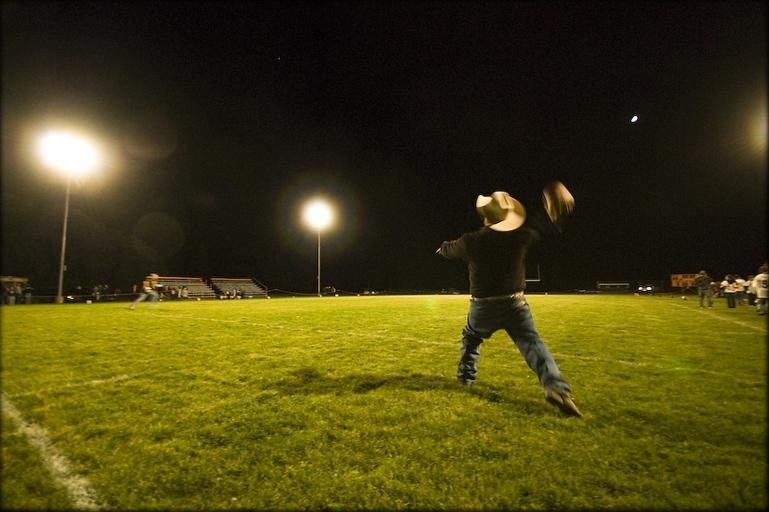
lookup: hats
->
[477,191,527,232]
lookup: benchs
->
[156,276,268,298]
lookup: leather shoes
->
[543,384,583,417]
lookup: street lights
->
[302,198,335,294]
[34,134,96,296]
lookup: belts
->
[469,291,523,301]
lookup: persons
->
[0,272,188,311]
[224,287,242,300]
[435,179,583,418]
[694,263,768,315]
